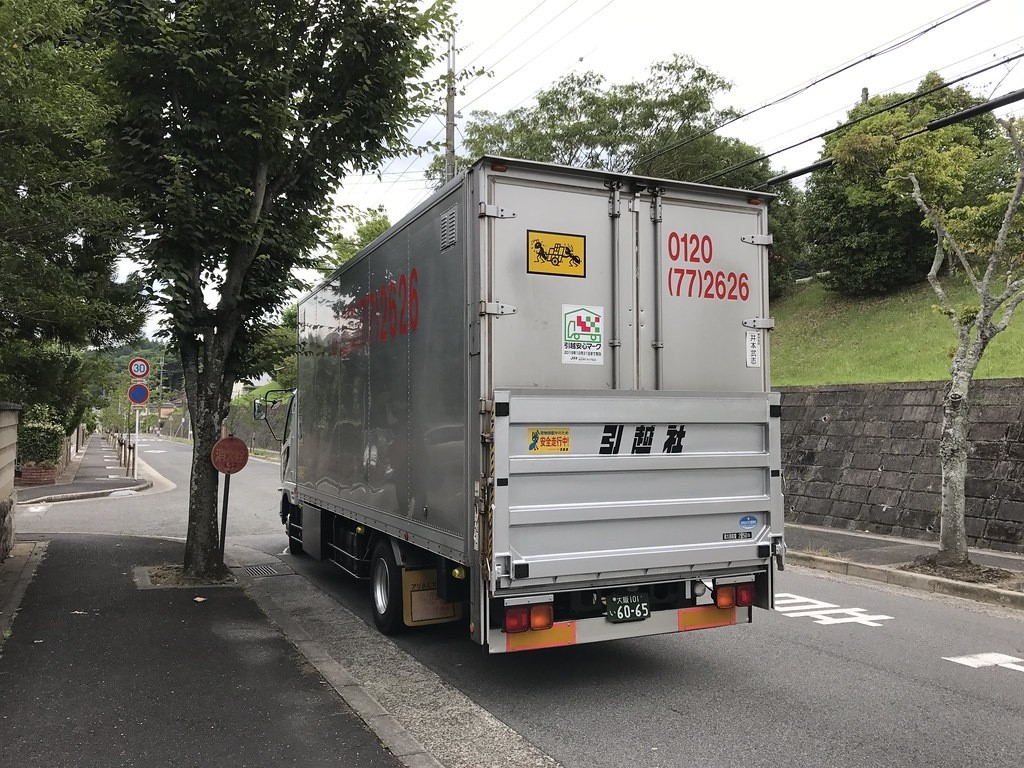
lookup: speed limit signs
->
[128,358,149,379]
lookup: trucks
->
[251,153,790,656]
